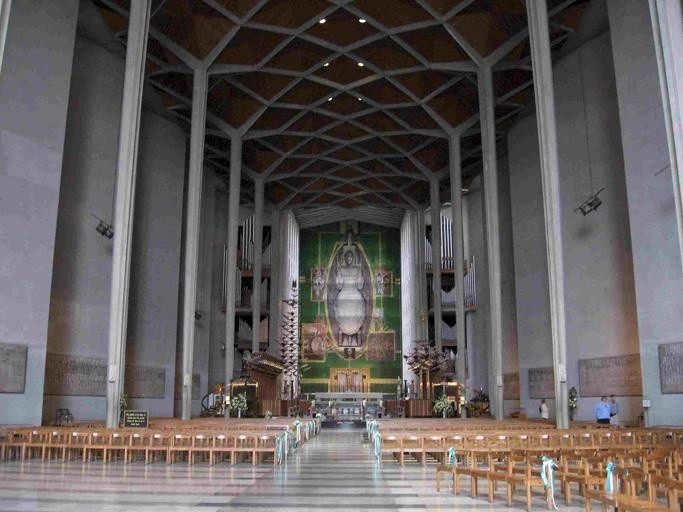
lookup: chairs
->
[365,418,683,512]
[0,404,322,466]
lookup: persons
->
[594,396,611,423]
[607,394,619,423]
[540,399,549,420]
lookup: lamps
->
[195,312,202,320]
[579,196,601,216]
[91,213,113,239]
